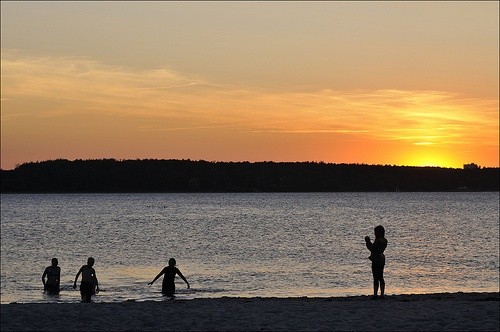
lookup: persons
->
[73,257,100,297]
[42,258,61,293]
[365,226,388,300]
[148,258,190,294]
